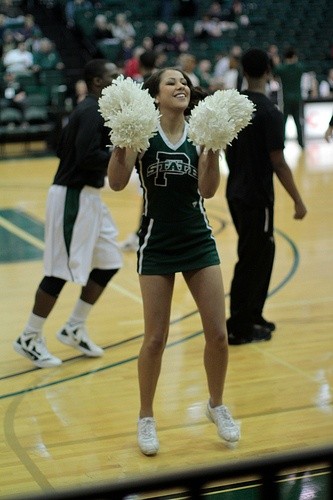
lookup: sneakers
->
[207,402,240,442]
[16,332,62,367]
[136,417,158,454]
[229,316,275,344]
[56,325,104,357]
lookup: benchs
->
[0,0,326,157]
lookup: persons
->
[12,58,121,369]
[107,68,240,456]
[0,0,333,151]
[223,48,306,346]
